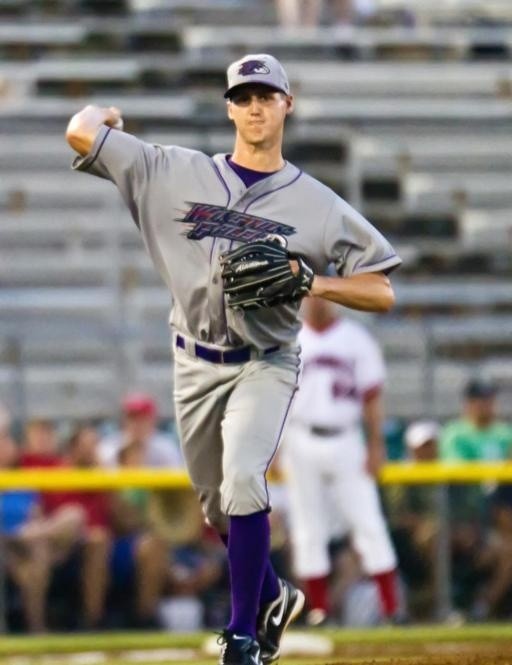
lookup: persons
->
[65,53,401,665]
[1,379,511,625]
[276,283,401,624]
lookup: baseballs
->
[114,118,123,130]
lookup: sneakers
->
[219,573,308,665]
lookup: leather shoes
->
[177,337,280,364]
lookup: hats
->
[223,52,291,99]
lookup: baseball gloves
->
[218,241,313,312]
